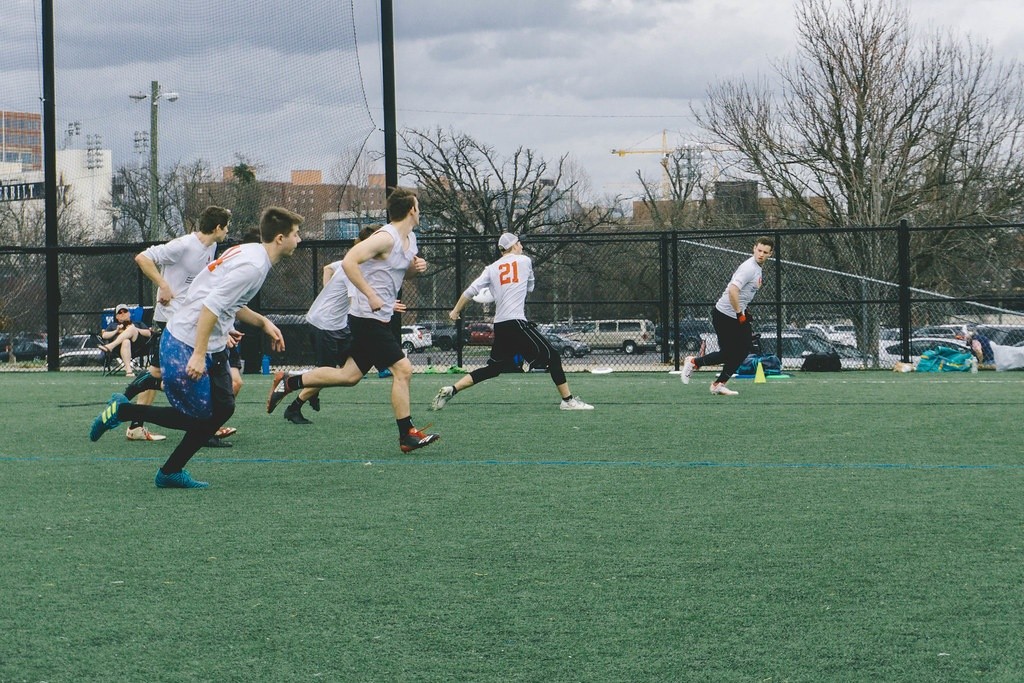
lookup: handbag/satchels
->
[801,347,842,372]
[735,353,782,375]
[917,346,975,373]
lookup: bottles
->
[972,356,978,374]
[262,355,272,374]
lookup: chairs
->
[101,307,159,376]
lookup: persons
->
[433,233,593,410]
[283,223,385,424]
[90,205,303,487]
[681,236,774,395]
[967,324,998,370]
[266,186,441,454]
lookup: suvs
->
[416,321,472,351]
[546,320,657,355]
[400,324,433,354]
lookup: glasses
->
[118,310,129,315]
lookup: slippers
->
[96,343,112,354]
[125,372,136,378]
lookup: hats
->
[498,232,526,251]
[116,303,129,315]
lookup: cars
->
[466,323,496,345]
[654,316,857,357]
[0,331,107,367]
[541,332,591,358]
[875,322,1023,369]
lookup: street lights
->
[128,81,181,313]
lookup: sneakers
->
[124,369,153,400]
[709,382,739,396]
[126,426,167,441]
[284,405,313,424]
[154,465,209,488]
[214,427,238,438]
[200,437,233,447]
[266,372,292,413]
[90,392,130,442]
[431,386,454,410]
[680,356,697,384]
[400,421,440,452]
[560,395,595,411]
[308,391,320,412]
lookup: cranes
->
[610,128,724,200]
